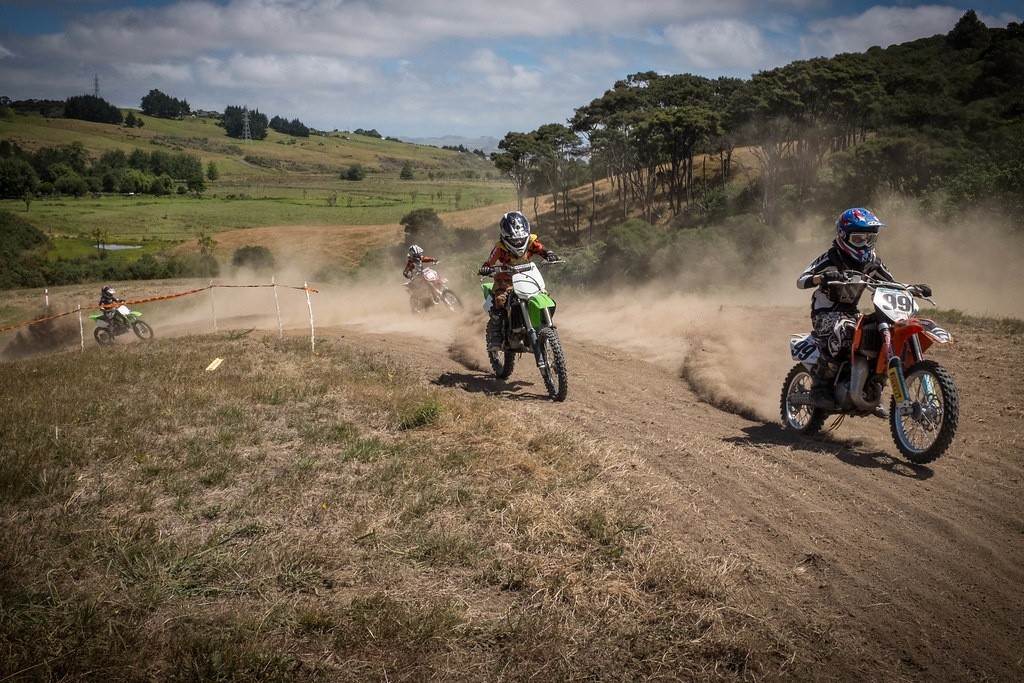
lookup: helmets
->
[836,208,887,255]
[101,285,115,299]
[408,245,424,260]
[500,211,531,258]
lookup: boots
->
[873,406,890,419]
[486,307,506,352]
[810,353,835,410]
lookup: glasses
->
[835,221,880,249]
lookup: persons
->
[402,244,440,302]
[99,285,126,345]
[796,207,933,413]
[479,210,562,352]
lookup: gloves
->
[406,274,416,281]
[820,271,844,287]
[914,284,932,298]
[432,258,439,264]
[545,253,561,263]
[478,267,492,276]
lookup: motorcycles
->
[476,255,570,403]
[87,301,154,347]
[779,268,960,467]
[400,260,465,315]
